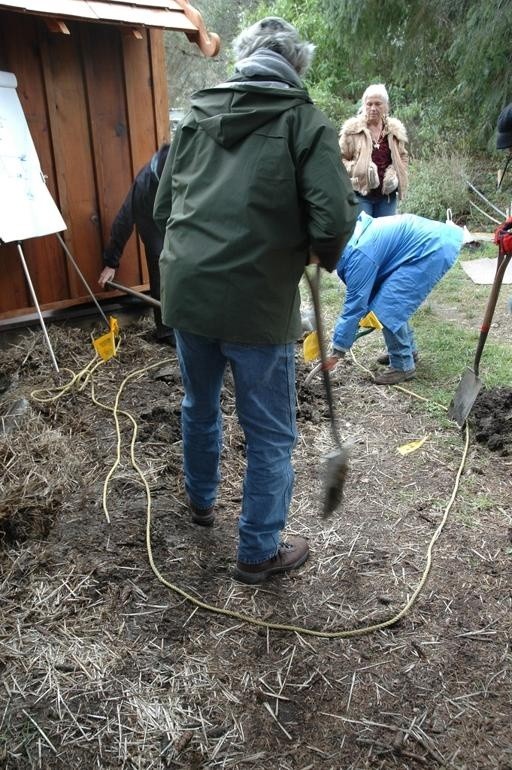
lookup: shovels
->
[302,262,352,521]
[446,231,512,431]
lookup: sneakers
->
[372,364,416,385]
[231,537,309,584]
[188,497,215,526]
[377,349,418,365]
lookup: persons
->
[151,16,359,584]
[338,83,408,219]
[319,210,465,384]
[97,143,177,348]
[494,102,512,255]
